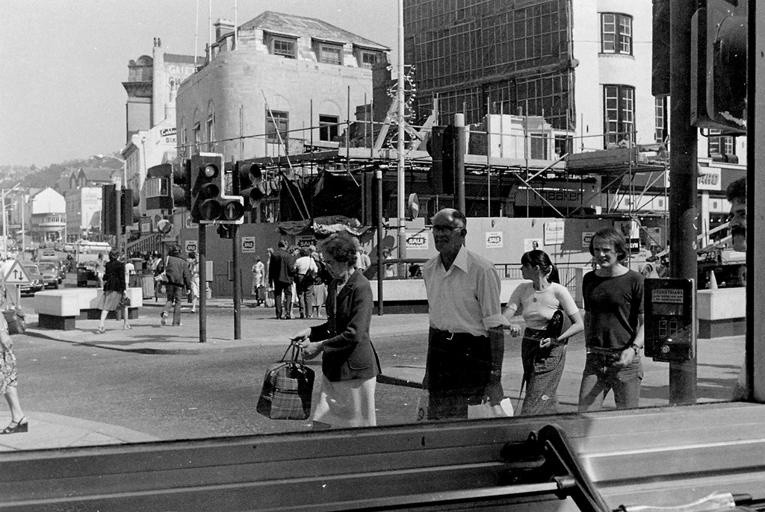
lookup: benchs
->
[33,287,142,331]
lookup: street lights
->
[16,192,39,252]
[94,152,130,197]
[0,180,22,249]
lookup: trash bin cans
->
[136,275,155,300]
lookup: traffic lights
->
[221,196,244,223]
[170,158,194,211]
[190,152,223,222]
[235,162,262,223]
[700,0,750,128]
[424,124,439,196]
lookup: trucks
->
[694,244,748,291]
[74,241,112,287]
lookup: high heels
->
[0,415,28,435]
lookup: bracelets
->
[490,361,503,367]
[631,344,641,355]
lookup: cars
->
[0,239,78,299]
[359,255,435,280]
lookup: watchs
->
[490,369,503,375]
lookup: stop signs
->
[410,194,419,219]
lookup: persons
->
[415,207,509,420]
[67,244,210,334]
[382,246,396,279]
[532,241,539,249]
[725,178,747,252]
[290,231,381,430]
[0,313,30,434]
[578,228,647,412]
[503,249,585,416]
[252,237,372,321]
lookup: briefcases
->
[255,284,267,300]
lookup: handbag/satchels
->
[545,311,574,345]
[256,340,315,421]
[120,292,130,306]
[466,396,514,420]
[305,268,323,285]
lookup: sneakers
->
[92,299,326,335]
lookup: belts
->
[429,326,489,343]
